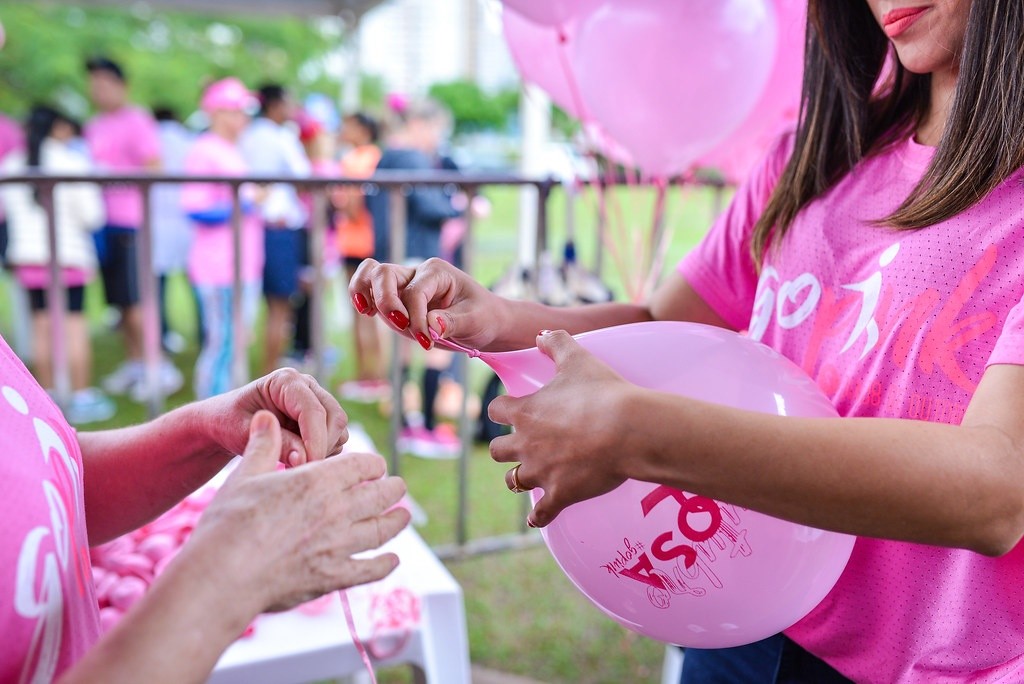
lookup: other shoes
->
[47,386,119,426]
[396,423,462,460]
[340,378,393,405]
[129,362,185,405]
[105,361,132,397]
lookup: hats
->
[388,92,412,112]
[201,78,263,110]
[40,86,89,130]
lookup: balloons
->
[496,0,808,184]
[429,322,854,646]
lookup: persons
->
[0,331,414,682]
[0,58,477,461]
[181,75,266,392]
